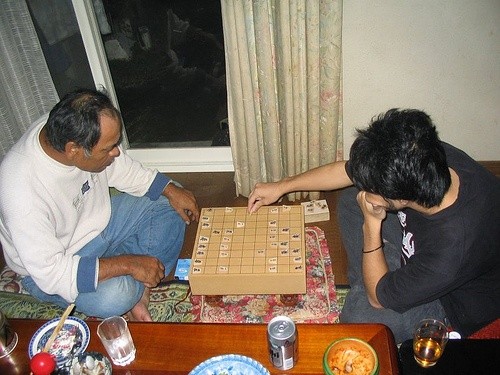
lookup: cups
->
[413,319,449,367]
[97,316,137,366]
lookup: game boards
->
[187,205,307,295]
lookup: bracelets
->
[362,244,383,253]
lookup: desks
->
[0,319,398,375]
[398,339,500,375]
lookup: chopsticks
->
[43,305,74,351]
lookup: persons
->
[0,86,201,322]
[247,108,500,344]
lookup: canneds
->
[266,316,299,371]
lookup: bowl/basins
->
[323,337,379,375]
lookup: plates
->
[58,352,112,375]
[28,316,91,368]
[188,354,271,375]
